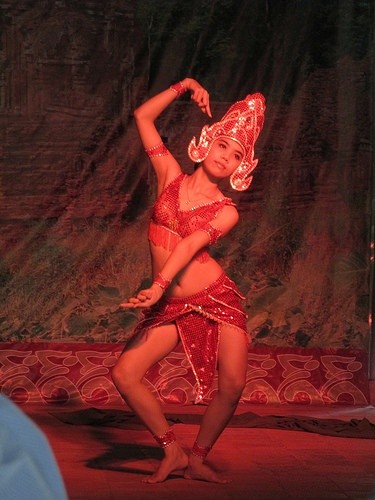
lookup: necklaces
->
[184,177,213,205]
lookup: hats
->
[187,93,266,192]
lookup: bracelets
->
[171,81,187,99]
[154,273,171,291]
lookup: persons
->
[112,78,265,485]
